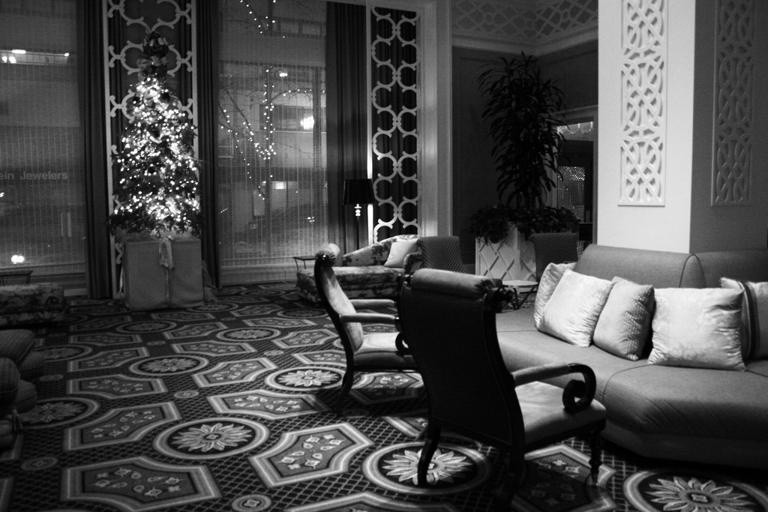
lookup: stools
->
[293,255,318,270]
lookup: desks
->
[0,269,34,285]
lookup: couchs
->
[494,244,768,468]
[296,235,422,306]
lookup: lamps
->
[342,180,379,250]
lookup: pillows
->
[531,263,768,370]
[383,235,418,270]
